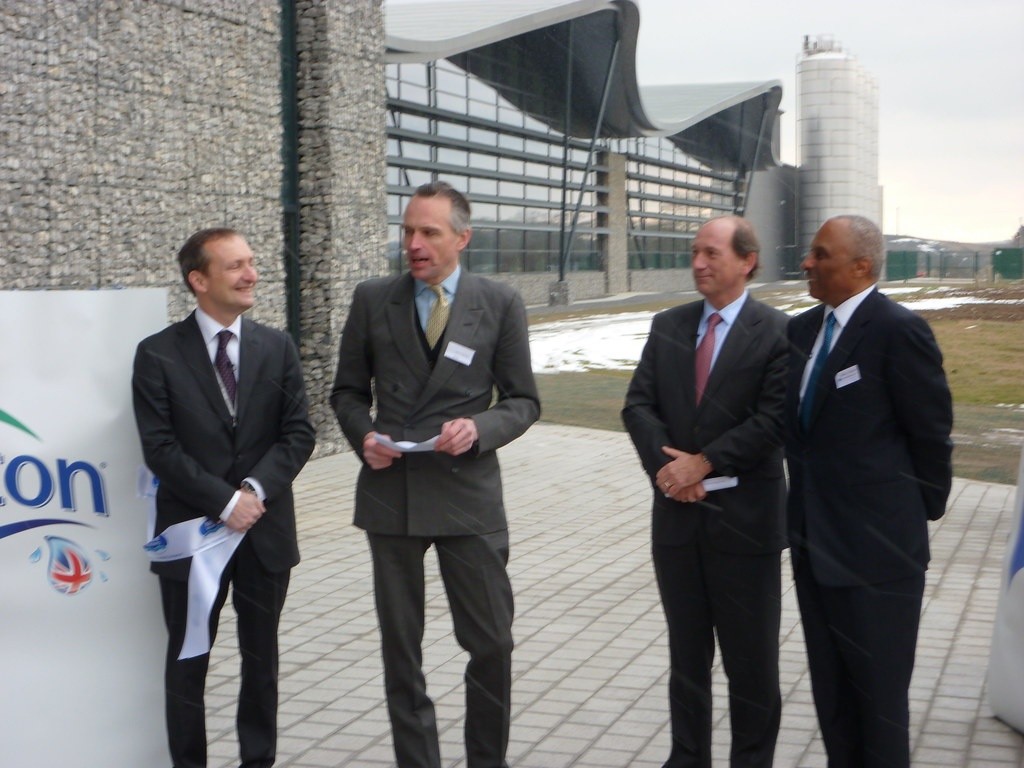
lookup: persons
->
[328,181,542,768]
[783,214,955,768]
[620,214,798,768]
[131,227,316,768]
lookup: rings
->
[663,481,672,488]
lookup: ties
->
[798,312,836,431]
[214,331,236,410]
[695,312,723,407]
[425,284,450,350]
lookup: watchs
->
[242,481,259,497]
[701,452,715,469]
